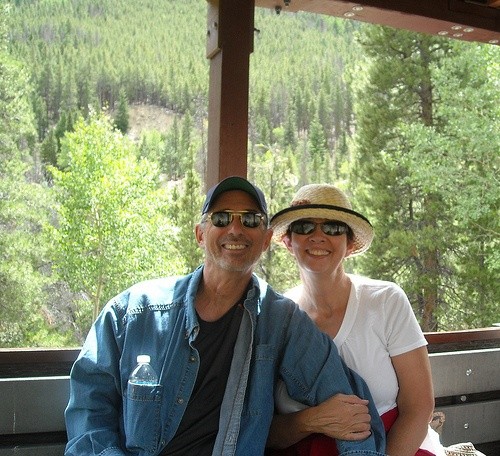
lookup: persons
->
[265,183,448,456]
[63,176,387,456]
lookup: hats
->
[270,184,375,259]
[202,176,267,218]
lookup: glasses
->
[205,209,265,228]
[289,220,349,236]
